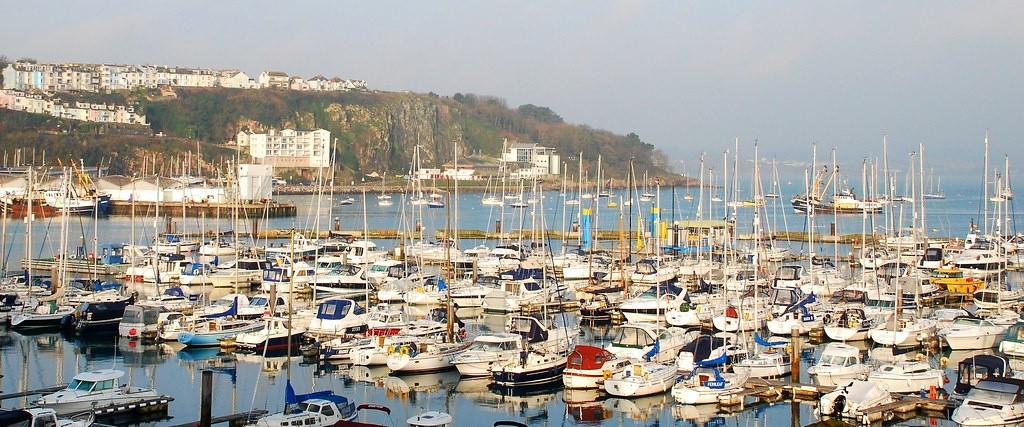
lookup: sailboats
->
[0,128,1024,427]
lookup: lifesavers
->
[188,323,195,332]
[386,345,395,355]
[603,369,613,381]
[399,346,409,356]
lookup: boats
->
[791,164,882,214]
[0,407,95,427]
[37,337,161,415]
